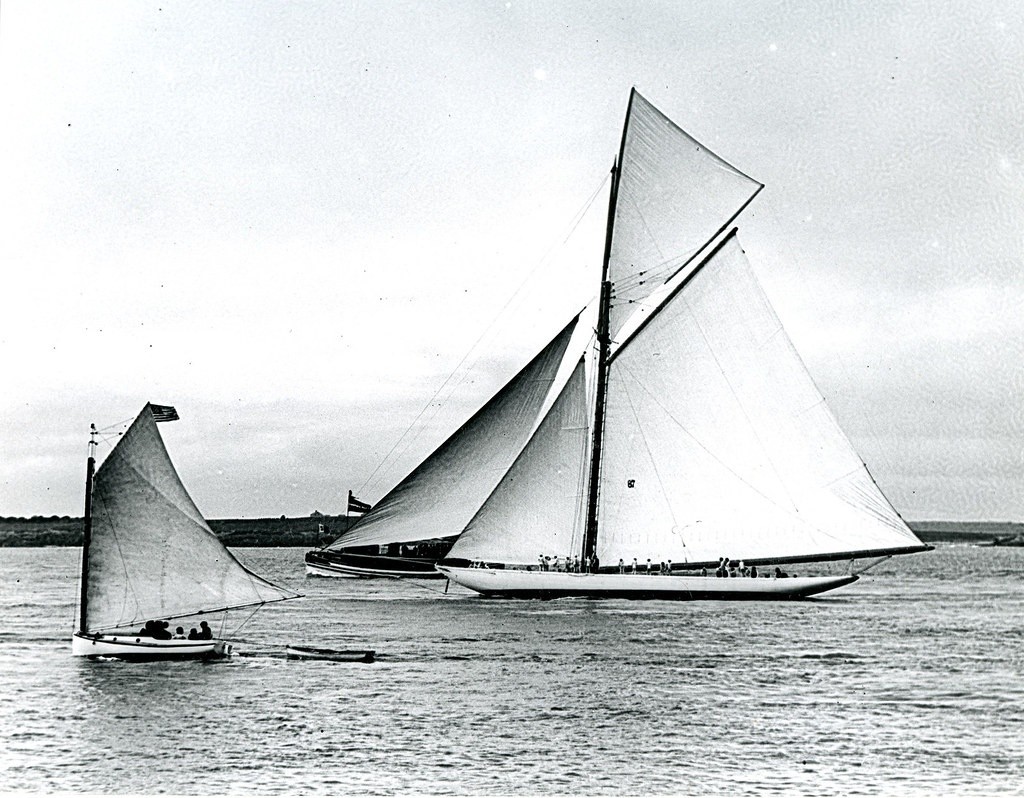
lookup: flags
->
[348,497,373,514]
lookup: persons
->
[141,620,213,641]
[393,541,448,556]
[535,552,795,579]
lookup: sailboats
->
[433,83,937,601]
[71,399,305,662]
[303,302,589,582]
